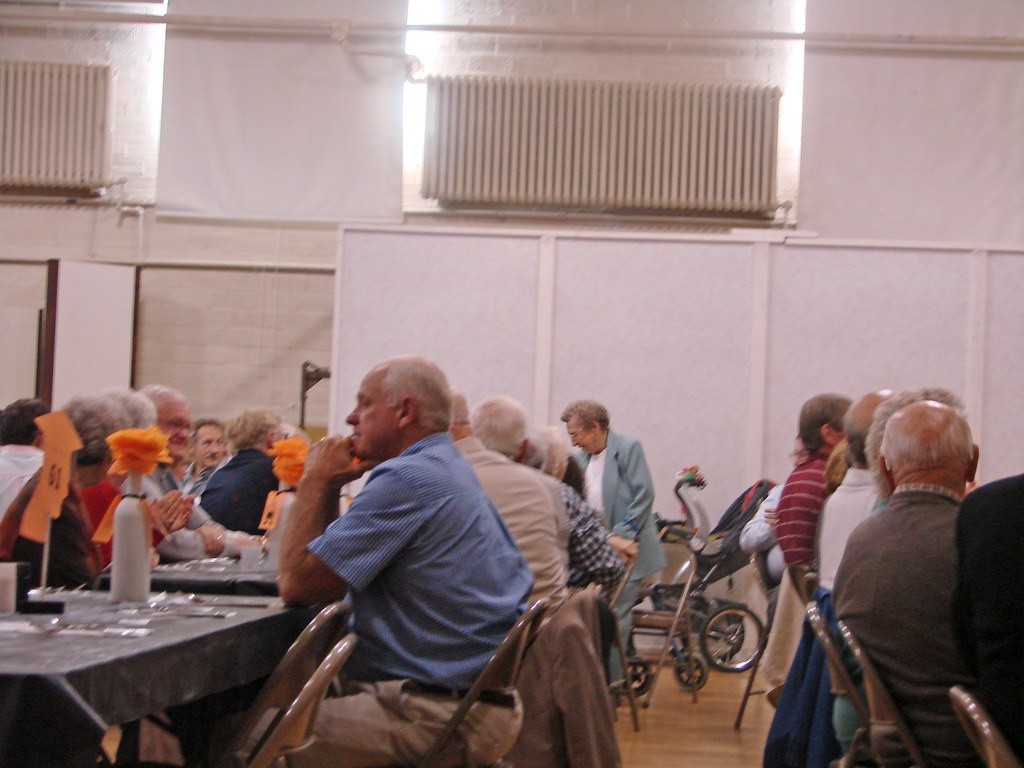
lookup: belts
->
[420,682,514,707]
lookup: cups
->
[1,562,17,618]
[241,546,261,573]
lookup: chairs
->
[212,542,699,768]
[734,552,1020,768]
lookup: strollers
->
[622,478,777,699]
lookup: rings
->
[320,436,326,442]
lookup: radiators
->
[0,58,114,196]
[418,72,782,221]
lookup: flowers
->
[265,438,315,490]
[105,425,175,476]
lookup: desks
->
[94,557,299,594]
[0,587,333,768]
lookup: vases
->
[112,472,151,604]
[274,478,298,545]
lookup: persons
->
[279,359,639,768]
[561,401,668,698]
[738,387,1024,768]
[0,384,309,614]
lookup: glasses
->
[156,420,196,439]
[569,420,594,439]
